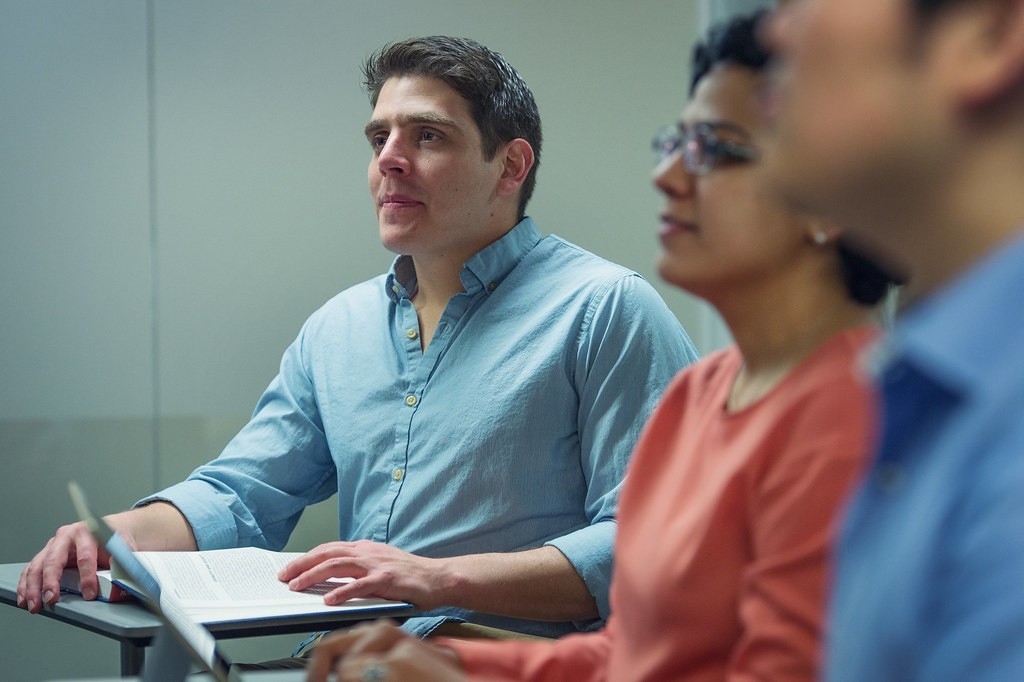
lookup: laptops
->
[65,481,342,682]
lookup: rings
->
[362,660,388,681]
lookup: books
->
[60,546,409,628]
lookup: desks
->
[0,563,418,682]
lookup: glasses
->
[648,120,758,177]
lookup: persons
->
[15,36,700,682]
[310,0,1023,682]
